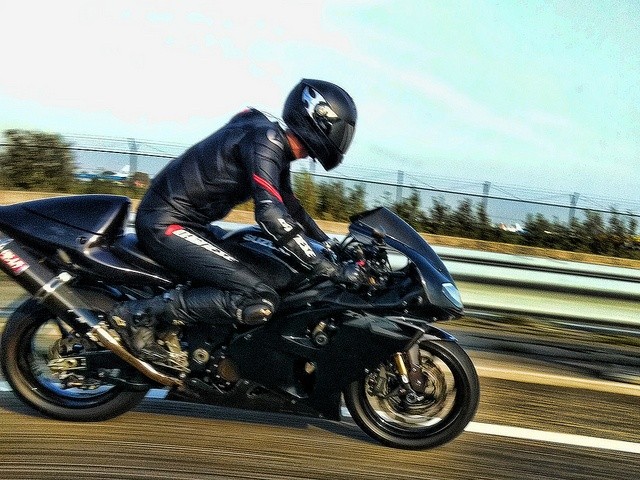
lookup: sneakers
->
[106,299,169,363]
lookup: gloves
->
[330,261,369,291]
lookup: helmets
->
[281,78,357,172]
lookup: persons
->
[110,79,370,362]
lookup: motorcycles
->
[1,191,480,450]
